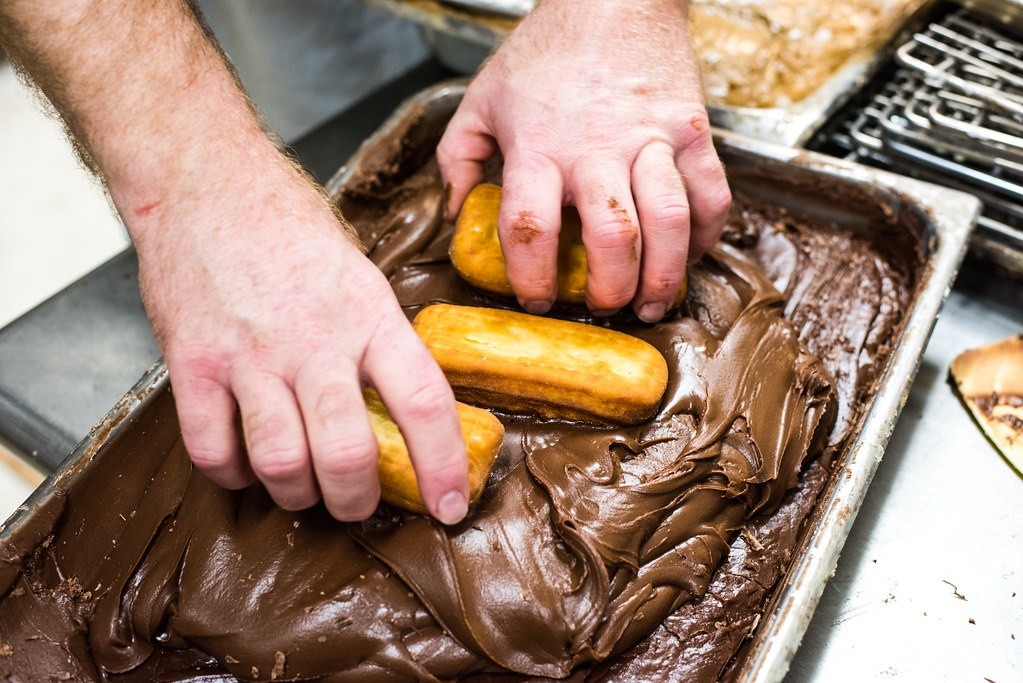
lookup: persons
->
[0,0,731,524]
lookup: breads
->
[359,387,506,518]
[409,305,668,429]
[446,182,591,303]
